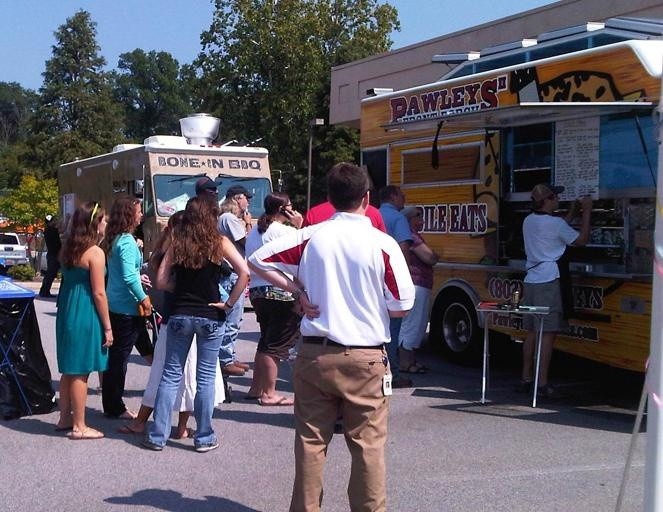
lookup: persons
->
[397,206,439,376]
[375,184,414,260]
[306,164,388,238]
[244,191,304,407]
[142,196,250,453]
[53,200,115,439]
[246,160,418,512]
[38,212,71,299]
[101,196,152,420]
[517,182,593,398]
[195,176,222,203]
[218,185,253,375]
[150,209,192,274]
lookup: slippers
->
[244,395,261,399]
[412,362,429,371]
[174,428,194,439]
[117,425,143,434]
[399,364,425,374]
[261,397,295,406]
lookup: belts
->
[302,335,384,350]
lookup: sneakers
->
[196,443,219,451]
[518,378,534,392]
[531,385,570,403]
[391,379,413,388]
[234,360,249,371]
[223,365,245,376]
[140,437,163,451]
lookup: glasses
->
[285,203,294,207]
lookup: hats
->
[226,186,253,199]
[196,177,223,194]
[531,184,565,202]
[45,215,55,223]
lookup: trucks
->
[57,113,273,312]
[360,16,662,363]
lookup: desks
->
[477,305,550,408]
[0,278,36,417]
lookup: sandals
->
[69,428,105,439]
[55,426,74,430]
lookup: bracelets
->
[244,222,253,228]
[292,285,304,300]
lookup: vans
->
[0,233,27,267]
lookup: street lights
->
[307,118,325,214]
